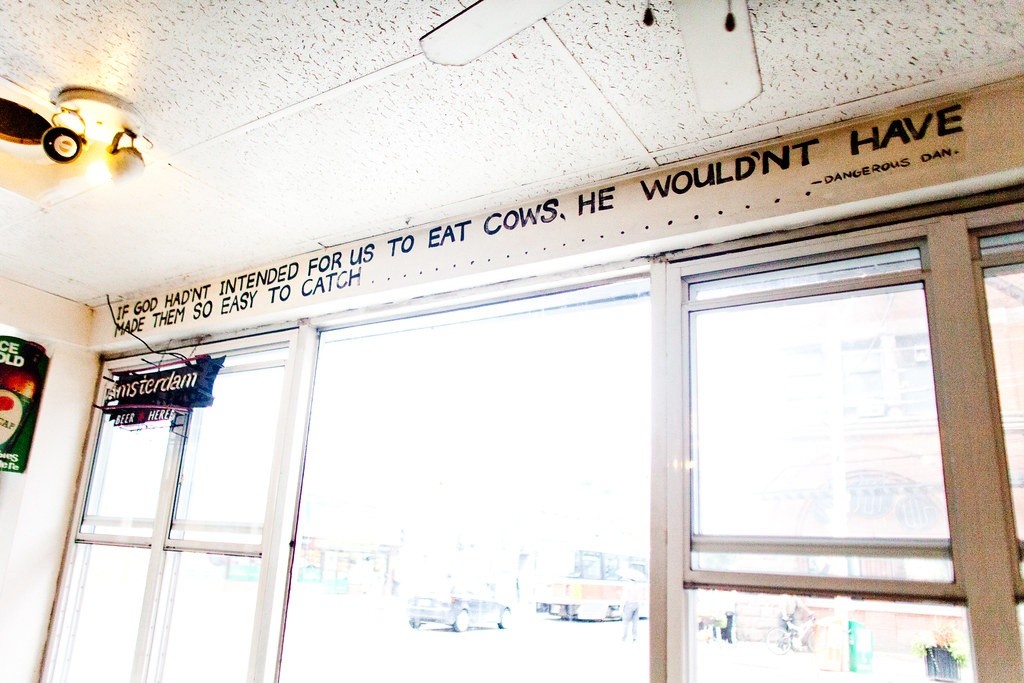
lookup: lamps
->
[41,87,154,172]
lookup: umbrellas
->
[615,567,648,582]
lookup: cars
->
[407,585,512,634]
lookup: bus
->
[535,548,651,621]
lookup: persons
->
[775,592,816,653]
[619,576,639,643]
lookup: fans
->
[418,1,763,115]
